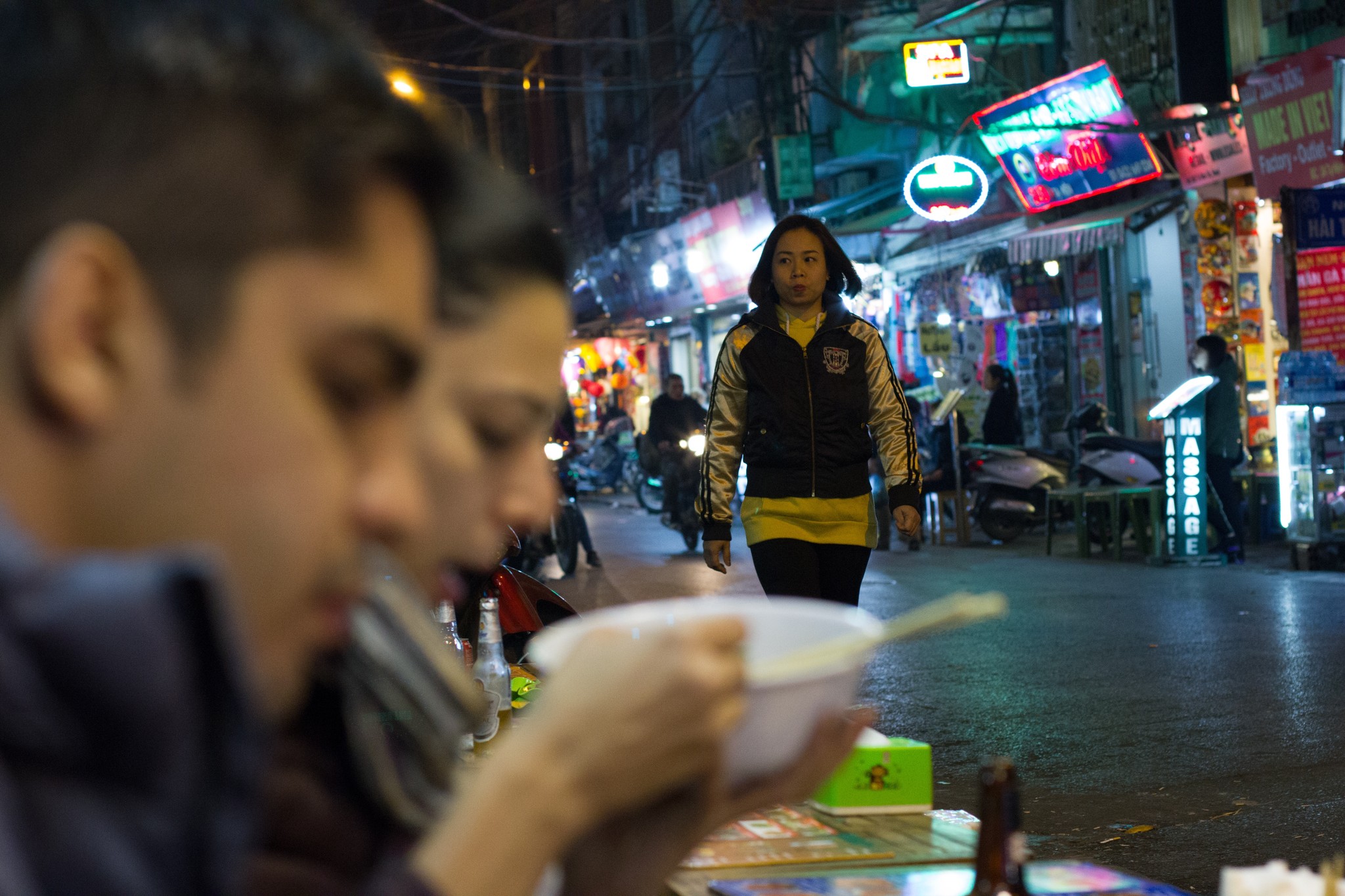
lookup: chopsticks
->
[751,588,1009,674]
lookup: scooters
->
[510,411,708,576]
[957,396,1163,545]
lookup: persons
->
[697,215,927,607]
[873,393,972,550]
[0,0,877,896]
[981,365,1026,449]
[1192,333,1246,563]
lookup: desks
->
[666,801,1184,896]
[1046,486,1164,560]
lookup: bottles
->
[432,600,465,666]
[967,761,1034,896]
[469,596,513,756]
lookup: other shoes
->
[586,552,601,567]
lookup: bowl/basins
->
[523,595,882,776]
